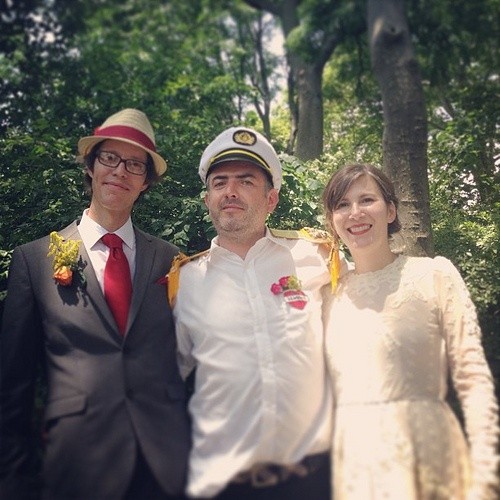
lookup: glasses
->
[93,150,149,176]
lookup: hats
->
[198,127,282,194]
[78,108,168,176]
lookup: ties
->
[100,233,132,339]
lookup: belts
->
[227,453,331,491]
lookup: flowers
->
[48,232,91,293]
[272,275,309,309]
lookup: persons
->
[316,162,499,500]
[0,110,194,500]
[163,126,356,500]
